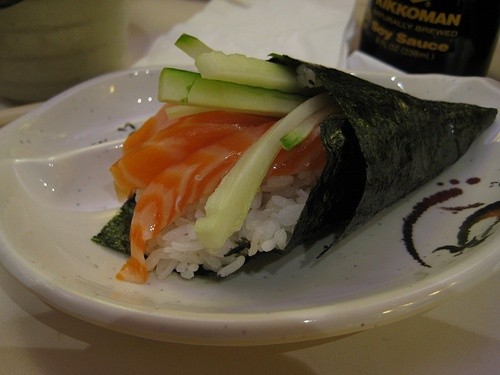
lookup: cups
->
[0,0,129,108]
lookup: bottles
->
[358,0,500,77]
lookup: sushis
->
[87,33,498,283]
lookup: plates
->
[0,64,500,348]
